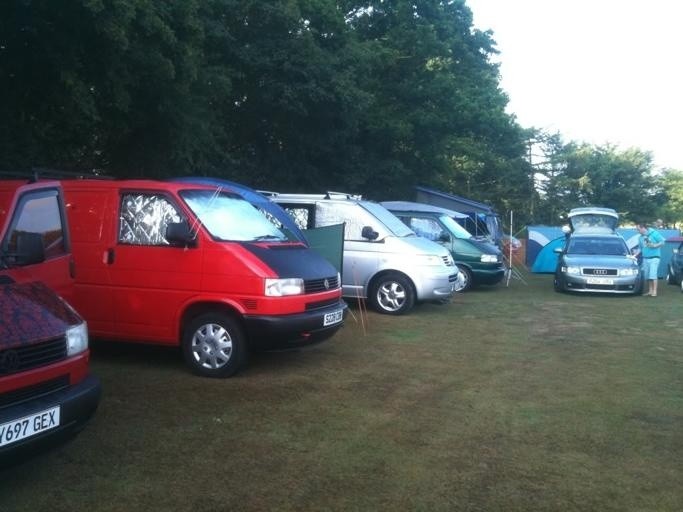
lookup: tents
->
[528,235,570,275]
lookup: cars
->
[552,207,643,296]
[665,241,683,292]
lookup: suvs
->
[388,208,505,293]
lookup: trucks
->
[415,186,503,259]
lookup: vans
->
[0,167,347,378]
[0,180,101,457]
[256,189,459,315]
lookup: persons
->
[631,222,665,297]
